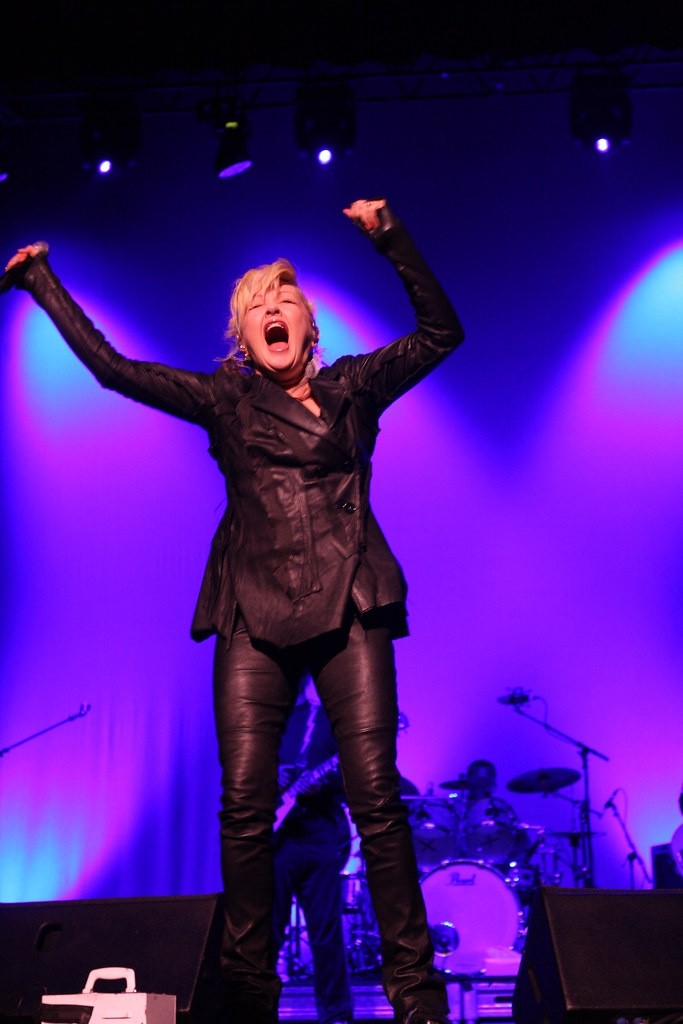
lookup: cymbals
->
[438,779,487,790]
[541,828,604,838]
[506,766,582,794]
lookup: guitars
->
[268,711,412,839]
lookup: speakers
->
[512,887,683,1024]
[0,891,226,1024]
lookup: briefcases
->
[41,967,177,1024]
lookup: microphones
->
[435,934,450,945]
[604,788,618,809]
[498,694,536,705]
[425,783,435,798]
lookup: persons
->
[0,197,466,1024]
[672,794,683,877]
[274,670,352,1024]
[455,759,529,847]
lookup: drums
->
[403,798,460,872]
[457,796,520,865]
[417,858,525,978]
[338,872,373,913]
[504,860,541,896]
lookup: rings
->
[366,201,371,206]
